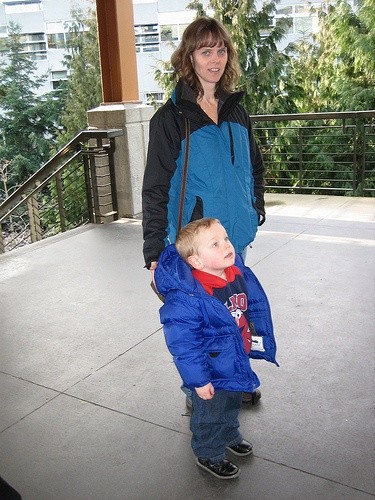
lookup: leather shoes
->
[227,438,253,456]
[195,457,241,479]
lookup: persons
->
[140,15,268,412]
[152,215,282,481]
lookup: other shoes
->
[243,388,261,402]
